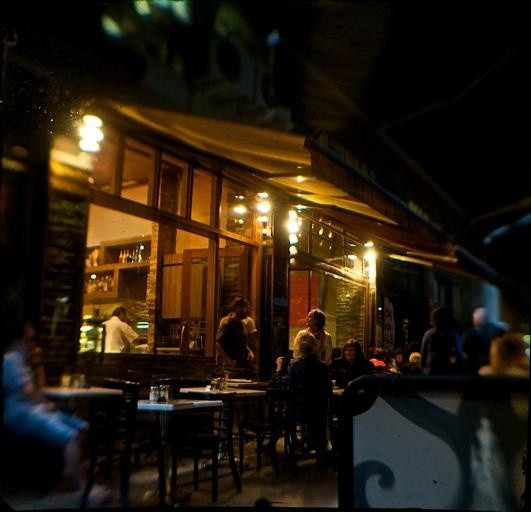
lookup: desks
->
[180,386,268,401]
[332,389,364,396]
[36,385,121,414]
[211,378,264,389]
[124,395,223,508]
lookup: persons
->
[274,332,331,451]
[216,300,255,379]
[328,338,373,387]
[482,338,530,376]
[371,344,426,377]
[460,309,507,369]
[99,306,148,359]
[2,316,111,505]
[305,307,333,366]
[420,304,465,376]
[215,298,260,384]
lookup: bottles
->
[86,274,112,291]
[118,242,144,263]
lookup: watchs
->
[33,363,45,369]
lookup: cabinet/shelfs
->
[83,235,152,306]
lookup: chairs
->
[170,389,245,498]
[288,415,329,477]
[238,388,299,472]
[80,380,180,505]
